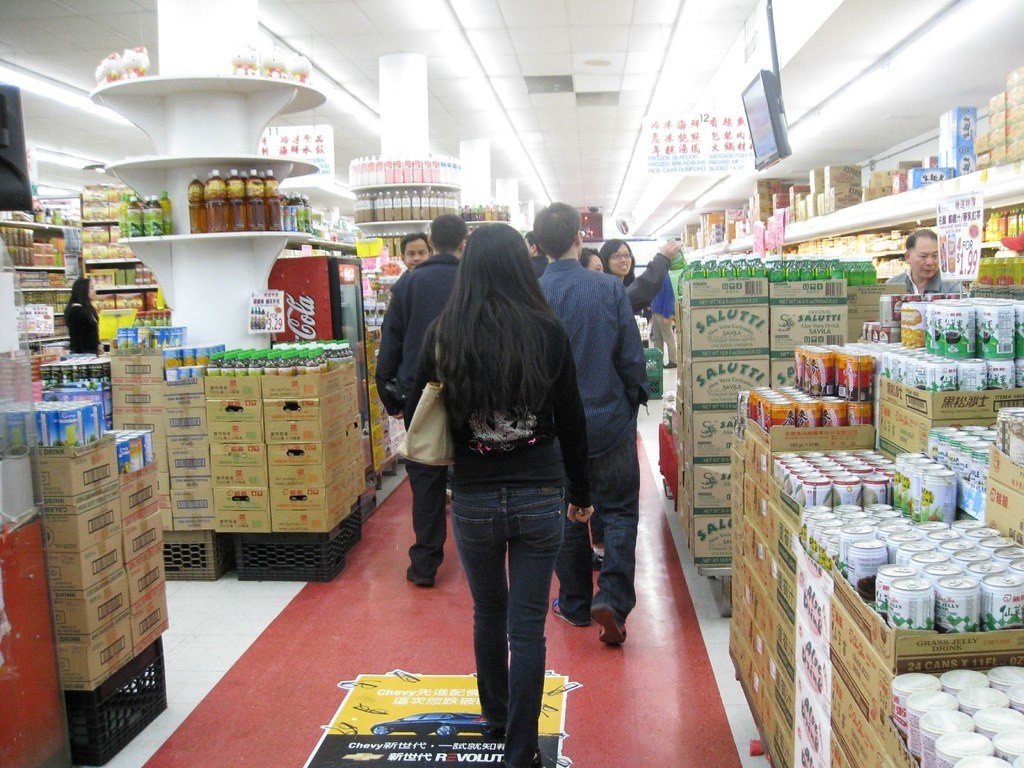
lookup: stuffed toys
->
[95,48,150,85]
[232,43,312,86]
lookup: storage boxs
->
[653,63,1024,766]
[12,183,361,767]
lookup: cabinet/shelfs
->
[85,74,327,251]
[0,219,86,343]
[72,217,168,294]
[349,183,463,238]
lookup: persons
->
[64,279,104,354]
[885,229,964,293]
[646,272,677,368]
[600,239,635,288]
[400,233,432,271]
[376,215,466,587]
[524,232,549,279]
[532,202,649,646]
[581,239,681,571]
[407,221,594,768]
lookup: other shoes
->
[488,720,506,736]
[664,361,678,368]
[590,550,604,571]
[406,566,434,585]
[503,748,541,768]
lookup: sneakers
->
[591,602,627,644]
[553,596,591,627]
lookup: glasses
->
[609,253,630,261]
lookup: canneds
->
[0,226,68,337]
[736,256,1024,767]
[0,324,225,474]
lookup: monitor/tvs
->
[0,83,33,213]
[741,68,792,171]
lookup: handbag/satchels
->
[397,322,454,466]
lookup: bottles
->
[245,170,282,231]
[363,232,405,257]
[188,175,209,233]
[384,154,438,183]
[119,191,173,238]
[207,340,353,376]
[384,191,430,221]
[353,192,385,223]
[281,192,314,233]
[363,256,407,326]
[439,156,464,187]
[982,209,1024,242]
[204,170,230,232]
[134,310,173,328]
[430,191,459,221]
[313,223,342,243]
[6,247,33,266]
[349,155,384,188]
[485,202,511,221]
[458,203,484,221]
[225,169,248,231]
[0,227,33,246]
[764,260,876,286]
[26,208,62,225]
[250,307,266,330]
[667,238,769,302]
